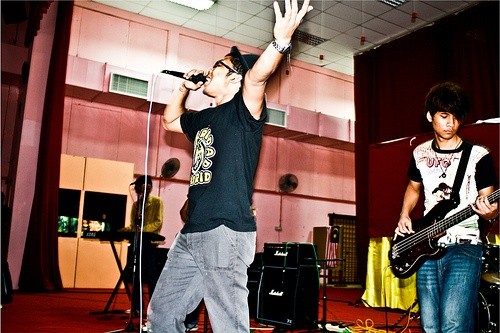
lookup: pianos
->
[81,230,166,318]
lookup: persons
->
[118,0,313,333]
[393,82,500,333]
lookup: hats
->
[232,45,261,71]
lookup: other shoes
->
[184,321,198,331]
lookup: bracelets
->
[488,213,498,223]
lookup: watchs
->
[271,38,292,54]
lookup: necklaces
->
[434,137,462,178]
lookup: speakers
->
[247,264,320,330]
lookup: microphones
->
[130,181,139,185]
[161,70,207,85]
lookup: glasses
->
[213,60,239,74]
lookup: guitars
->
[388,188,500,279]
